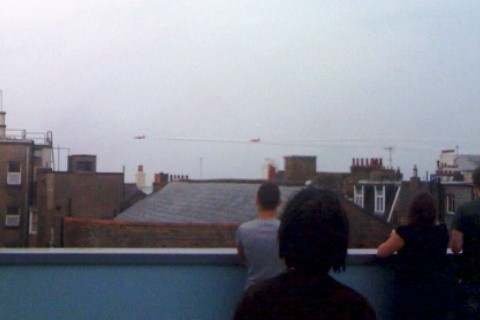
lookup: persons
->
[236,184,288,289]
[449,167,480,320]
[231,189,377,320]
[378,191,450,320]
